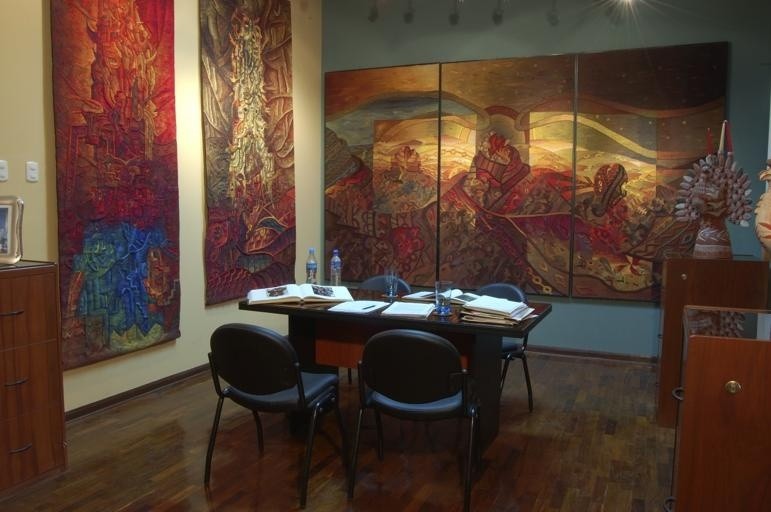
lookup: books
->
[462,294,535,325]
[422,288,481,305]
[381,301,435,319]
[247,283,354,306]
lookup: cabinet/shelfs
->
[0,260,72,502]
[651,253,770,429]
[668,300,770,512]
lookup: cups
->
[435,281,454,314]
[385,267,400,296]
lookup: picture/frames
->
[0,193,27,266]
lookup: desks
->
[233,289,554,463]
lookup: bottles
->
[306,247,318,284]
[330,250,343,286]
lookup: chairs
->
[344,330,491,511]
[343,274,415,390]
[194,322,349,511]
[465,282,536,415]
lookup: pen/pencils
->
[363,305,374,310]
